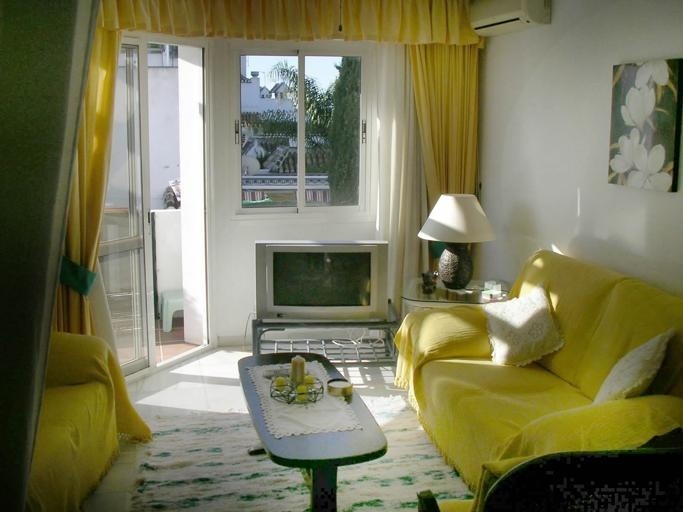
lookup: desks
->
[251,303,401,368]
[238,353,388,512]
[399,277,512,309]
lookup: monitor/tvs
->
[255,240,388,323]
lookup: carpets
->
[130,395,476,512]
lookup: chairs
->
[417,448,683,512]
[23,328,118,512]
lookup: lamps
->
[416,192,496,290]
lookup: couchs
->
[404,249,683,492]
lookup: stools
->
[161,288,184,332]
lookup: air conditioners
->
[470,0,551,38]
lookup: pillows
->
[592,327,677,405]
[481,281,565,367]
